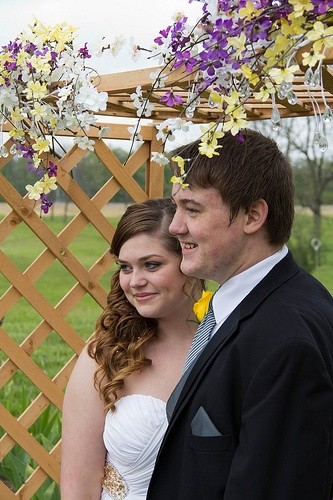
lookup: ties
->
[182,287,221,377]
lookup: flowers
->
[193,290,217,320]
[122,0,332,198]
[0,16,107,219]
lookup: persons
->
[146,129,333,500]
[59,198,208,500]
